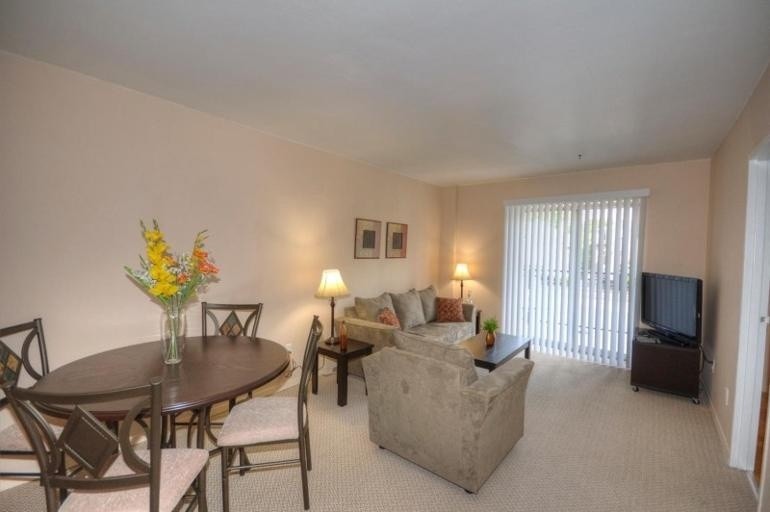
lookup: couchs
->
[363,328,531,495]
[331,285,476,381]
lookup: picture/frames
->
[353,218,382,259]
[385,222,408,258]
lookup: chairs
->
[217,317,322,511]
[0,372,211,511]
[0,320,117,503]
[157,302,266,479]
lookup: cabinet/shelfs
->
[626,329,704,402]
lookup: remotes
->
[640,331,648,337]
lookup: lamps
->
[449,261,474,300]
[315,268,352,346]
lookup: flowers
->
[123,215,222,358]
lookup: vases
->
[162,305,184,372]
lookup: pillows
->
[436,297,465,324]
[354,291,391,328]
[379,307,401,331]
[393,329,478,373]
[416,282,439,325]
[390,285,425,331]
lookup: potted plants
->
[482,316,500,347]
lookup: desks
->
[26,329,294,511]
[311,333,374,407]
[457,330,533,375]
[475,309,482,335]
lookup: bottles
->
[340,320,348,352]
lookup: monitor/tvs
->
[641,272,702,346]
